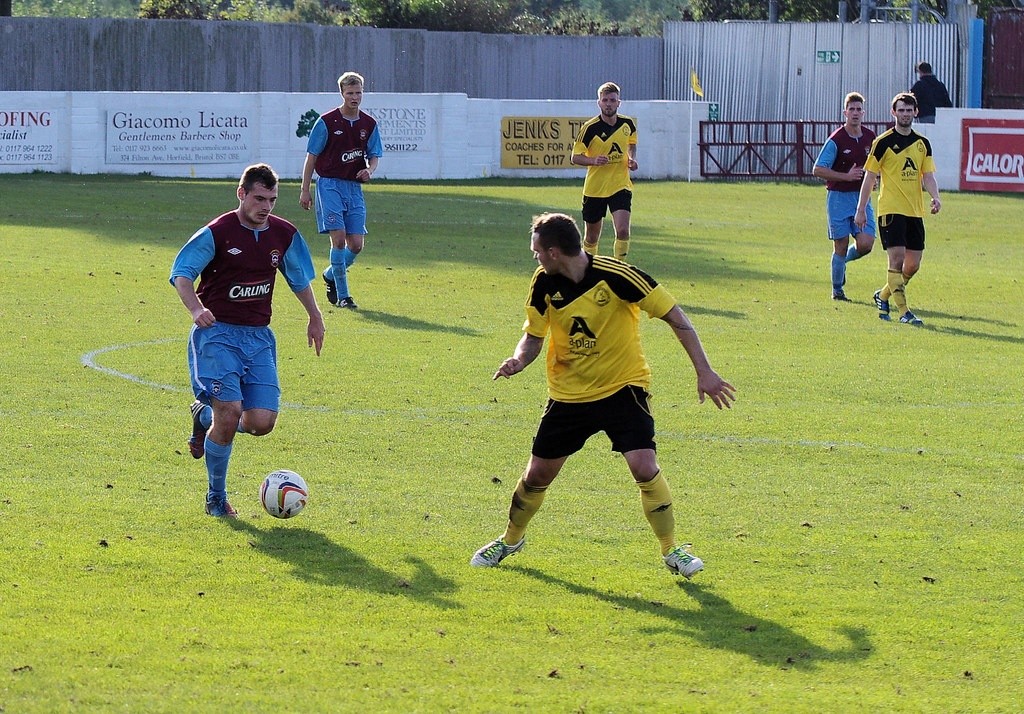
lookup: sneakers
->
[188,400,213,459]
[204,493,235,518]
[323,272,338,304]
[899,310,923,326]
[663,543,704,580]
[873,291,890,321]
[468,535,525,568]
[336,297,357,309]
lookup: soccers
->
[260,470,309,519]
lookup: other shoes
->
[832,291,853,302]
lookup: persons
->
[298,71,383,308]
[812,92,881,303]
[913,61,954,123]
[853,92,942,328]
[570,82,638,262]
[469,210,737,580]
[168,162,326,514]
